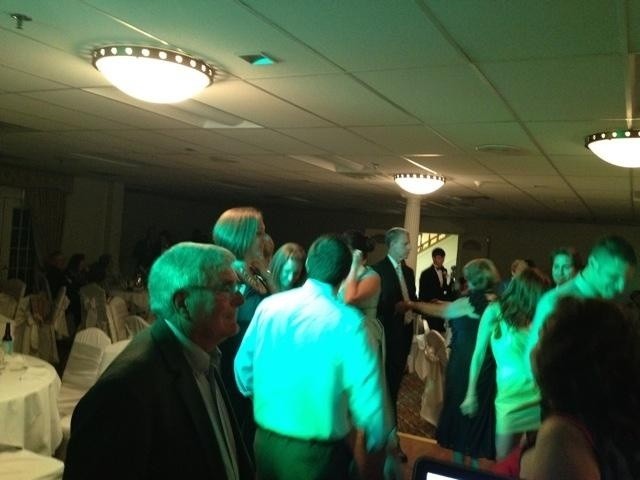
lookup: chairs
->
[406,313,449,425]
[0,268,154,480]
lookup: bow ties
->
[436,266,443,270]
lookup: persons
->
[233,234,396,479]
[47,206,305,349]
[550,247,579,286]
[519,295,640,480]
[420,248,454,332]
[337,230,387,480]
[459,268,554,463]
[371,228,418,410]
[502,259,534,291]
[409,257,502,461]
[529,236,637,349]
[61,239,256,480]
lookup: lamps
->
[584,130,640,169]
[91,45,215,104]
[393,173,446,195]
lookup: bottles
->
[119,272,129,291]
[2,322,14,354]
[136,271,142,288]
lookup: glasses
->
[191,280,246,296]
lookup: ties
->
[205,366,240,480]
[398,266,413,324]
